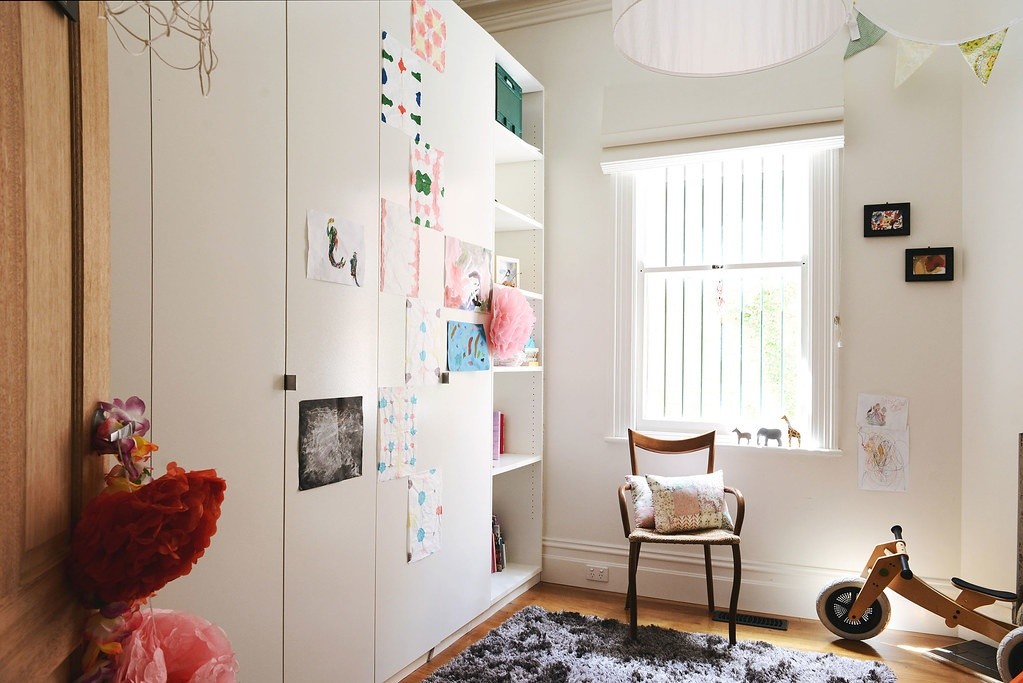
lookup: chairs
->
[615,427,747,646]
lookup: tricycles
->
[816,525,1023,683]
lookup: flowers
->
[483,285,537,358]
[78,609,243,683]
[65,466,229,621]
[89,394,161,472]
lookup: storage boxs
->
[495,61,523,140]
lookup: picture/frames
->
[905,246,956,283]
[864,200,911,238]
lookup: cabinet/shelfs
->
[107,0,548,683]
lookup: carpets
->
[419,604,898,683]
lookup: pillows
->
[644,468,734,534]
[624,473,655,529]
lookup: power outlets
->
[584,564,610,583]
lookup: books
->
[493,410,505,460]
[491,514,506,573]
